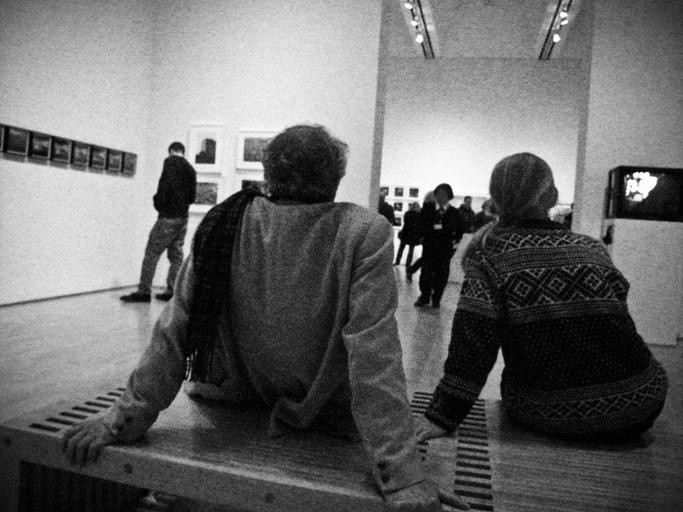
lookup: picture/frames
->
[233,132,271,170]
[0,123,136,177]
[235,175,274,198]
[184,177,222,215]
[379,184,420,232]
[187,126,223,174]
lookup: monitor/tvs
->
[607,165,683,224]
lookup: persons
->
[415,154,668,446]
[379,184,573,309]
[120,142,196,302]
[62,124,471,512]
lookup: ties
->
[437,207,445,215]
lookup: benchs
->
[0,366,682,511]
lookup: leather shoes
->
[415,294,439,307]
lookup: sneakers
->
[120,292,150,303]
[156,292,173,300]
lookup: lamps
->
[536,0,571,64]
[394,1,436,61]
